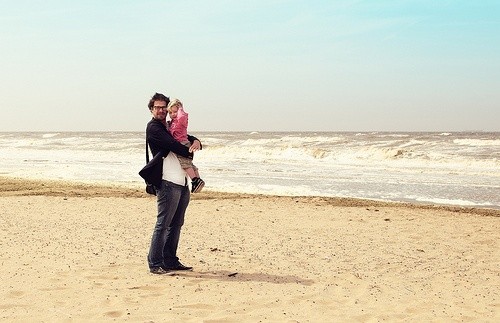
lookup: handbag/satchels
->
[144,180,157,196]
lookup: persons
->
[166,98,205,193]
[138,93,203,274]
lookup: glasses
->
[153,105,167,111]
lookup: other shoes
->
[151,266,170,274]
[190,178,205,193]
[168,259,194,271]
